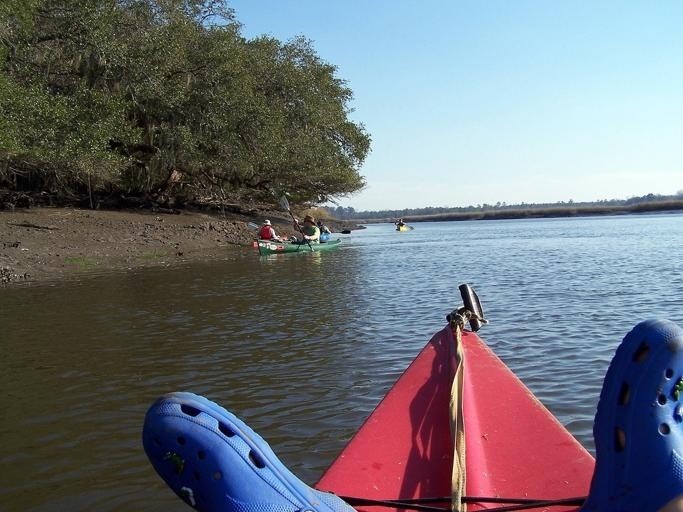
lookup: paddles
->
[248,222,260,230]
[280,195,315,253]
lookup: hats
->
[263,219,272,226]
[303,214,317,226]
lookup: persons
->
[397,218,405,226]
[292,214,320,245]
[255,219,280,243]
[316,220,331,234]
[141,317,682,512]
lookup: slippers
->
[140,389,358,511]
[583,317,683,511]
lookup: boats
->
[340,229,352,235]
[257,237,345,259]
[319,231,330,245]
[395,225,409,232]
